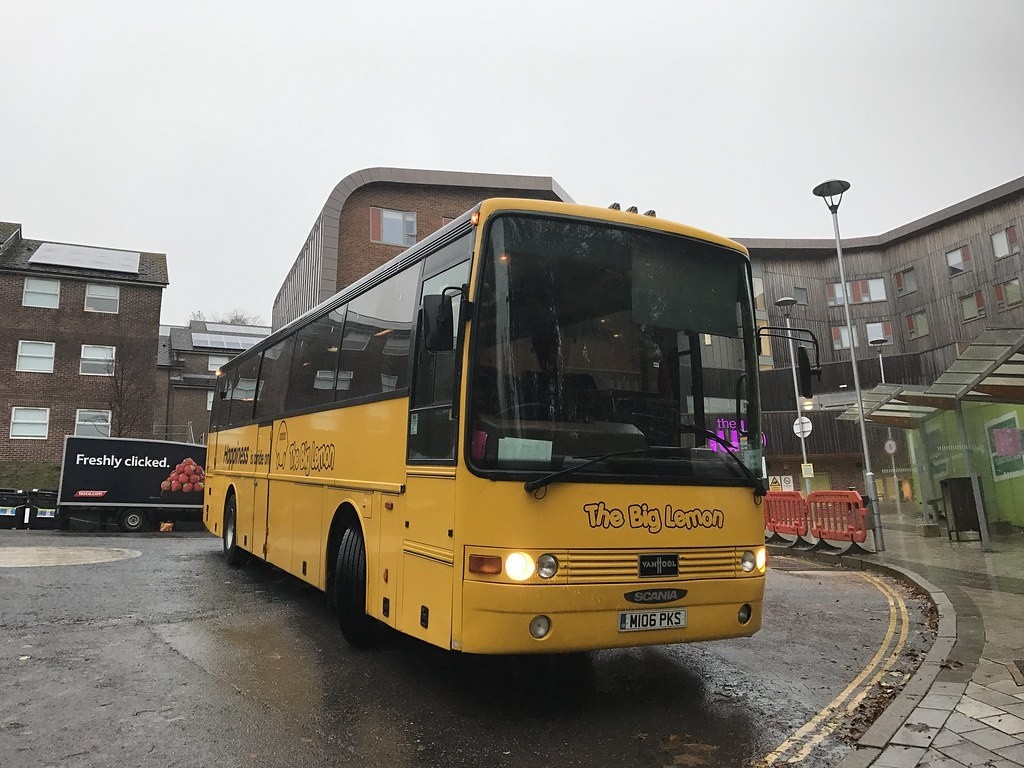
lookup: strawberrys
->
[161,458,205,492]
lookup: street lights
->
[812,178,885,555]
[775,295,811,496]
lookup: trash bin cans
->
[939,476,984,542]
[0,488,28,530]
[847,495,872,530]
[29,487,60,530]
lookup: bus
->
[203,199,770,654]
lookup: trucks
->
[55,434,208,533]
